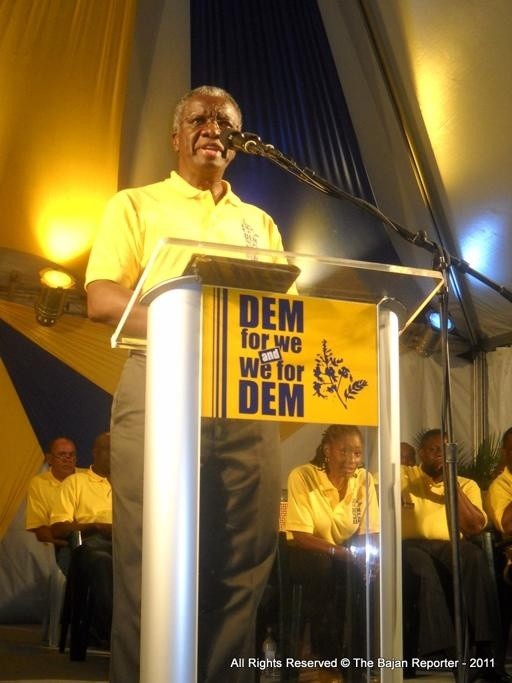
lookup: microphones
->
[220,125,277,160]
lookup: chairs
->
[482,521,503,602]
[274,531,308,677]
[57,531,93,661]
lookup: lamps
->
[33,268,75,327]
[415,310,453,358]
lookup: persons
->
[286,424,512,683]
[85,86,298,683]
[25,432,112,662]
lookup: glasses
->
[52,455,79,464]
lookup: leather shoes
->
[451,655,512,682]
